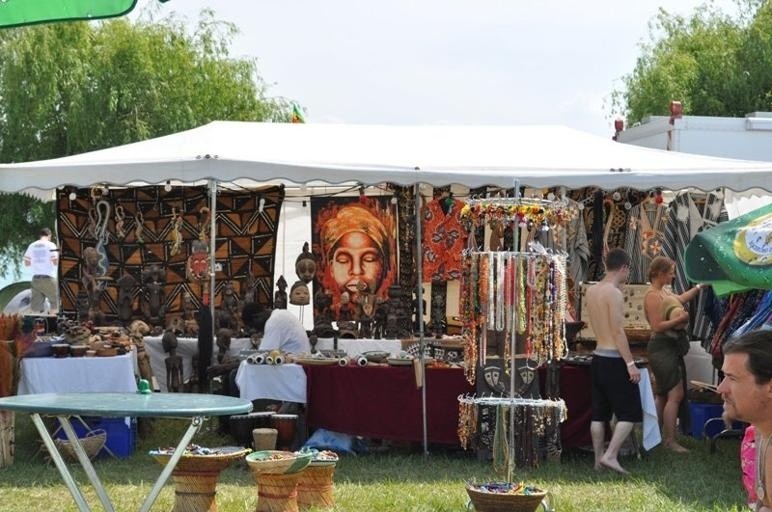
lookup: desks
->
[2,392,257,510]
[236,353,648,450]
[13,338,138,462]
[141,336,462,392]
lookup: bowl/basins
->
[388,359,413,366]
[52,344,97,358]
[24,338,64,356]
[579,337,597,350]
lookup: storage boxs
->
[685,400,730,440]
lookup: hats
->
[658,295,689,340]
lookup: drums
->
[271,414,299,451]
[230,415,253,448]
[248,411,276,449]
[252,428,278,451]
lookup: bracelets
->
[696,284,700,289]
[626,361,634,367]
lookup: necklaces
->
[454,196,570,455]
[755,434,771,501]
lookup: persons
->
[204,301,312,451]
[716,330,771,511]
[585,249,642,474]
[24,228,59,313]
[316,285,390,336]
[272,238,318,309]
[645,257,706,454]
[322,205,394,315]
[84,248,258,390]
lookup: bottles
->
[355,354,368,367]
[339,357,352,367]
[246,351,292,365]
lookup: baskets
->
[244,449,313,475]
[295,461,336,509]
[148,446,252,471]
[254,471,305,512]
[56,428,109,464]
[169,471,219,512]
[465,482,549,512]
